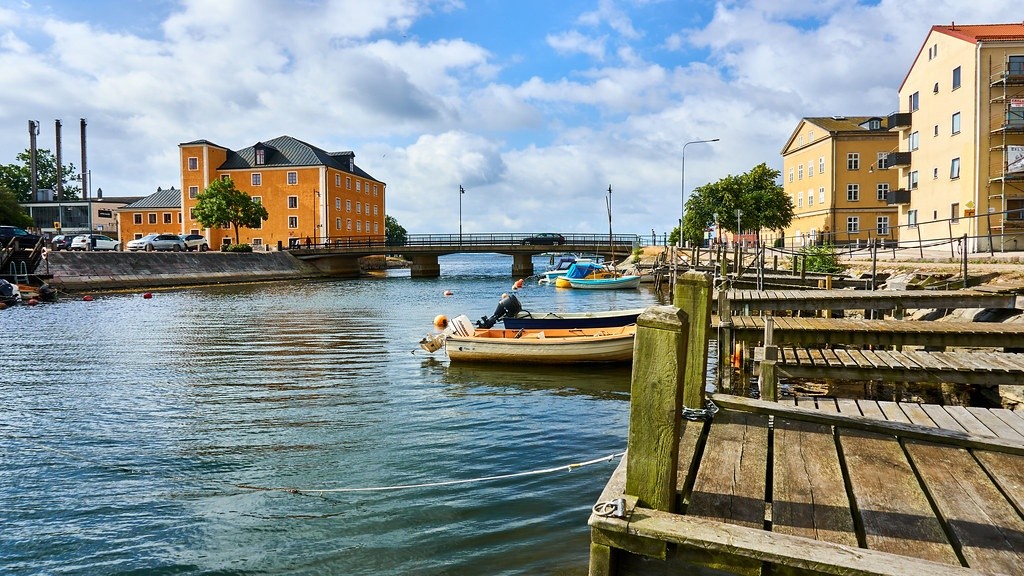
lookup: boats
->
[0,277,59,307]
[417,292,646,367]
[541,253,619,287]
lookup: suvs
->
[51,234,81,251]
[177,234,209,252]
[0,225,45,251]
[70,233,119,251]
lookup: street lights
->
[738,209,745,266]
[459,184,464,246]
[606,184,614,245]
[679,138,720,249]
[78,171,105,251]
[313,188,322,252]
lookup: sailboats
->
[556,195,643,290]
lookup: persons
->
[325,236,329,246]
[306,236,312,249]
[292,238,300,249]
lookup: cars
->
[126,233,186,252]
[521,233,565,247]
